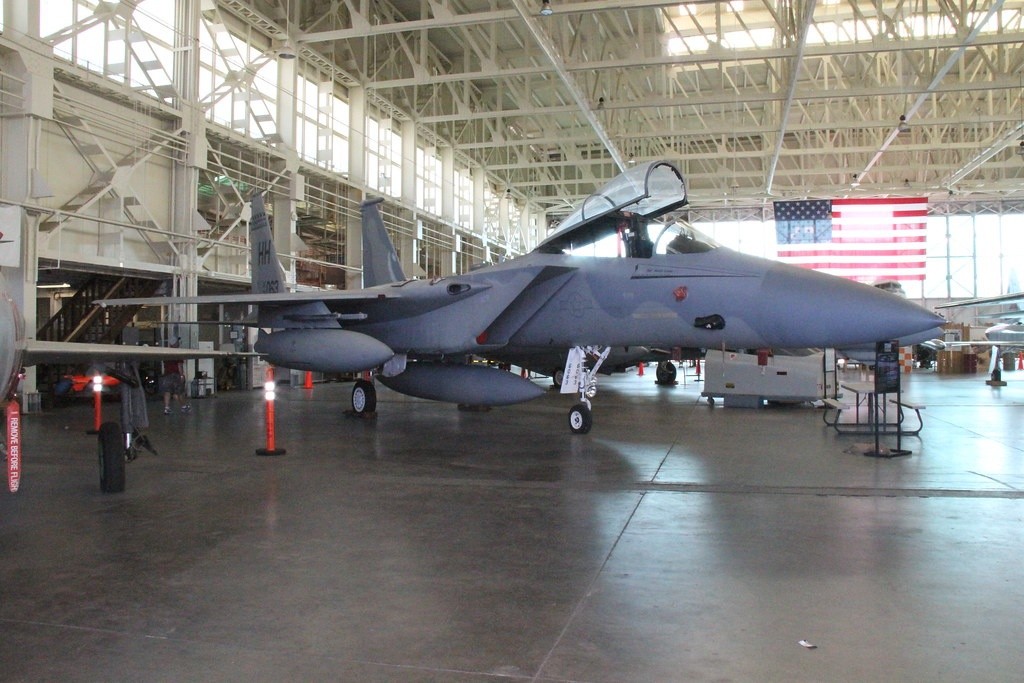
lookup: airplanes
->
[934,291,1024,341]
[0,235,272,460]
[92,160,953,433]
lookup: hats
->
[170,335,181,345]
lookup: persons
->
[161,337,191,415]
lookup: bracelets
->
[181,375,184,378]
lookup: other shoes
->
[164,407,172,413]
[181,405,191,412]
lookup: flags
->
[774,198,926,281]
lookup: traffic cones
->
[1017,350,1023,369]
